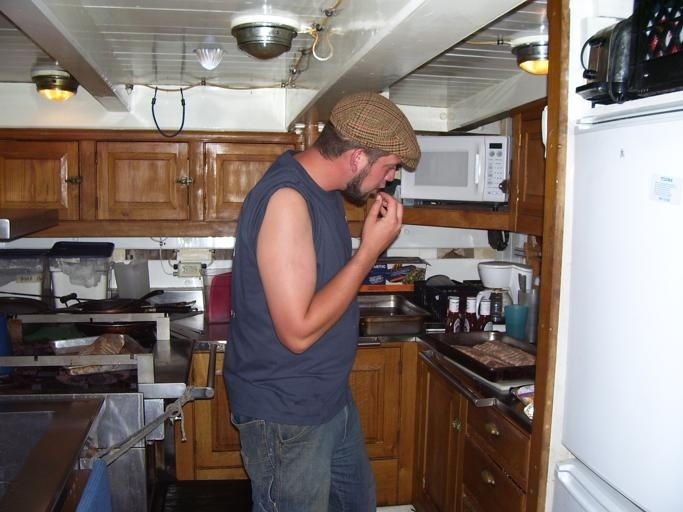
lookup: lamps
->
[229,8,299,61]
[30,56,79,103]
[509,34,549,75]
[192,47,227,71]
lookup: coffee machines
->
[475,262,534,334]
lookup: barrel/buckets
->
[112,255,151,299]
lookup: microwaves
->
[402,134,510,203]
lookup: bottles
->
[518,291,538,343]
[445,292,493,334]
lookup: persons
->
[224,92,421,512]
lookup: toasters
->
[573,15,632,108]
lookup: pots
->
[60,289,199,336]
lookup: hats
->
[329,89,421,174]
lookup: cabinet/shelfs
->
[174,343,418,508]
[416,354,469,511]
[512,101,548,236]
[0,128,88,236]
[466,400,529,512]
[88,129,200,239]
[200,131,295,238]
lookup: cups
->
[504,304,528,341]
